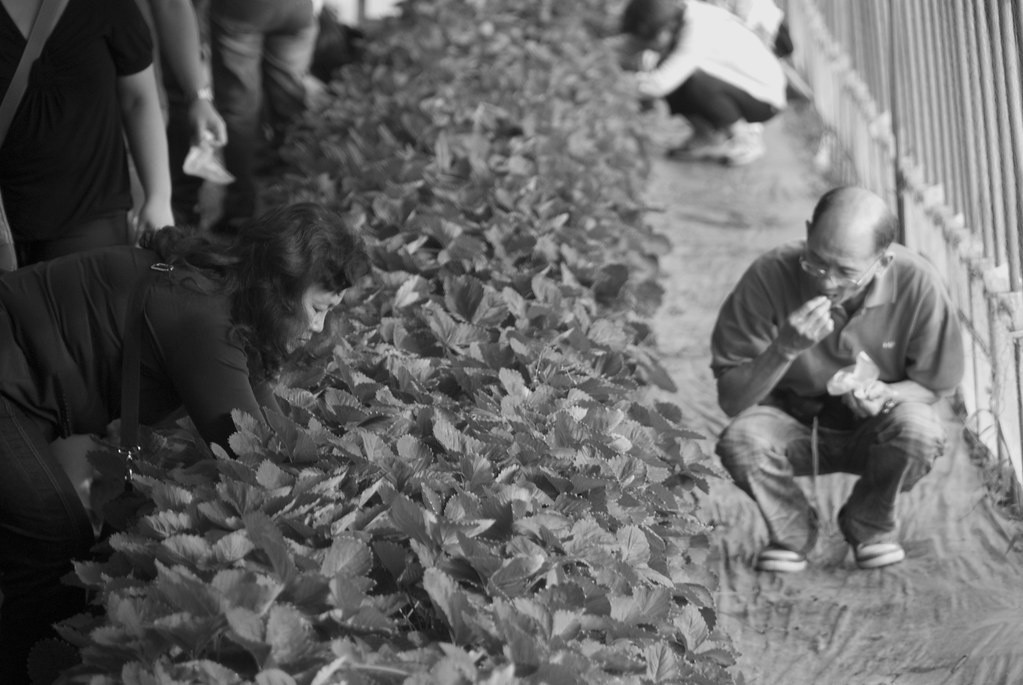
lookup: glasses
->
[799,246,883,290]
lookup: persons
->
[0,201,371,685]
[601,0,793,166]
[708,185,965,572]
[0,0,176,268]
[123,0,401,249]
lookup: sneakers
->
[756,507,819,571]
[837,510,904,569]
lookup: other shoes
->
[724,130,766,166]
[209,215,249,234]
[671,131,727,163]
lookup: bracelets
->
[883,384,898,414]
[185,86,214,105]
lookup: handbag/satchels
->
[86,486,157,622]
[775,25,792,57]
